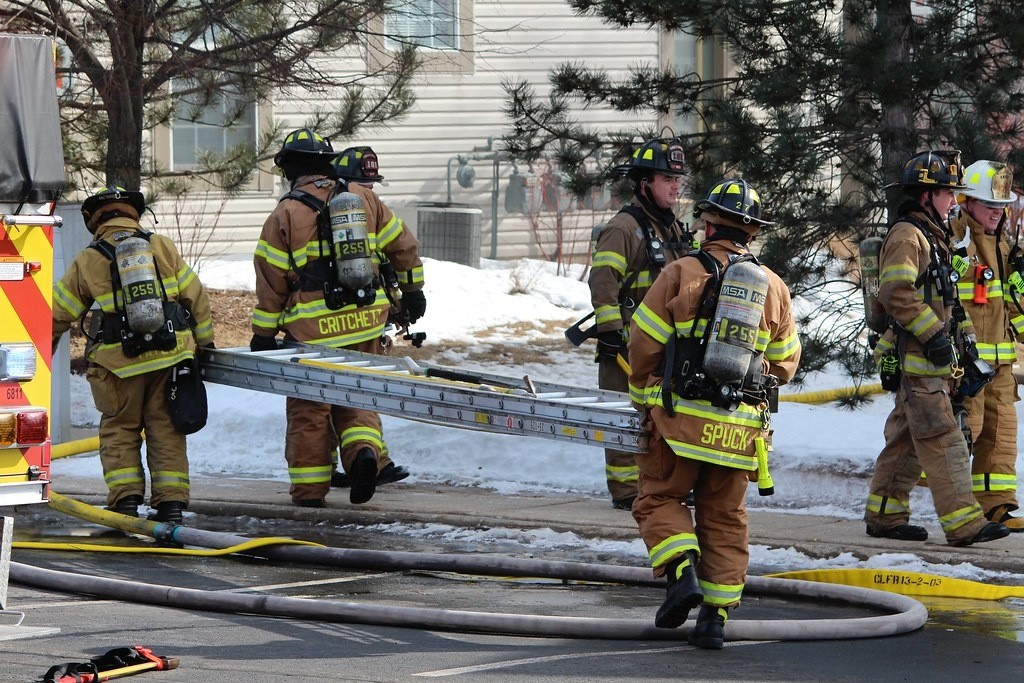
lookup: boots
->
[688,603,729,649]
[654,552,704,629]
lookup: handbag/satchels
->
[164,355,208,435]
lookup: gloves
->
[924,332,952,367]
[597,331,623,361]
[250,334,278,352]
[401,290,426,324]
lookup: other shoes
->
[350,448,378,504]
[988,505,1024,532]
[682,491,696,509]
[613,496,638,512]
[950,521,1010,547]
[292,497,322,507]
[866,521,928,541]
[376,467,409,486]
[330,471,350,487]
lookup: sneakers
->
[103,495,140,517]
[147,502,183,524]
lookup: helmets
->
[270,128,332,167]
[81,184,145,234]
[949,160,1017,203]
[882,149,968,190]
[694,178,778,230]
[331,146,384,180]
[614,137,690,177]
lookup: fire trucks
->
[0,30,77,631]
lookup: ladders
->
[196,336,649,454]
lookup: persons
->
[863,150,1011,545]
[250,129,426,508]
[588,137,697,510]
[942,161,1024,532]
[331,146,409,488]
[627,180,802,649]
[52,186,214,550]
